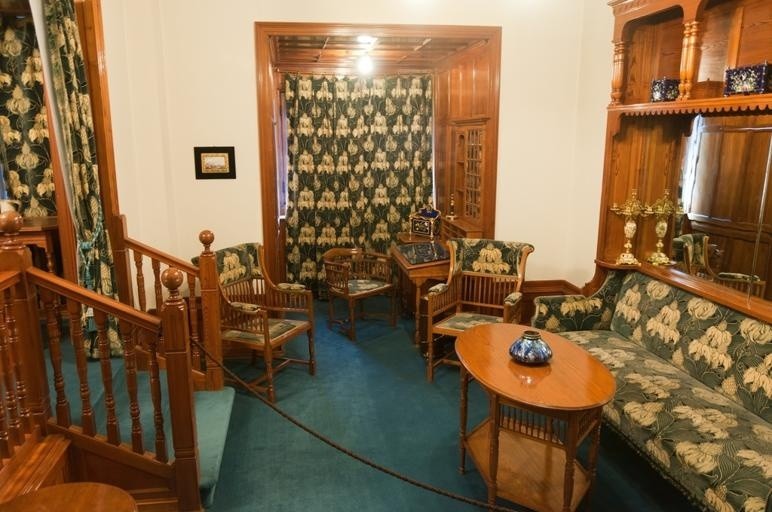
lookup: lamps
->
[647,189,676,266]
[609,189,654,267]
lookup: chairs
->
[682,232,767,299]
[322,248,397,341]
[427,237,534,383]
[190,242,316,403]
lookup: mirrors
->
[632,112,772,302]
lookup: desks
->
[454,323,616,511]
[390,213,483,346]
[0,215,70,320]
[1,481,138,512]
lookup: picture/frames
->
[194,146,236,179]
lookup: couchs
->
[531,270,772,512]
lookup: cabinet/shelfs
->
[607,0,772,116]
